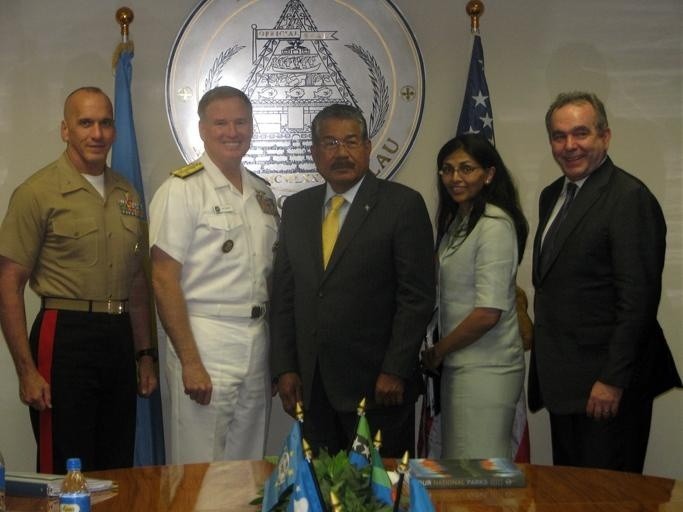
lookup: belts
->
[43,297,130,314]
[187,302,270,319]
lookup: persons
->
[147,85,280,463]
[268,103,438,459]
[524,92,683,474]
[423,134,530,466]
[1,87,158,475]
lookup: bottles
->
[0,453,5,512]
[59,459,91,512]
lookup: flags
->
[109,31,174,465]
[261,398,438,511]
[417,33,542,458]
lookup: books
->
[398,453,525,494]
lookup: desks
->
[0,460,683,512]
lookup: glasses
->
[439,164,481,174]
[313,135,366,150]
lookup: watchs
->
[135,348,155,360]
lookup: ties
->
[323,194,344,271]
[541,182,578,261]
